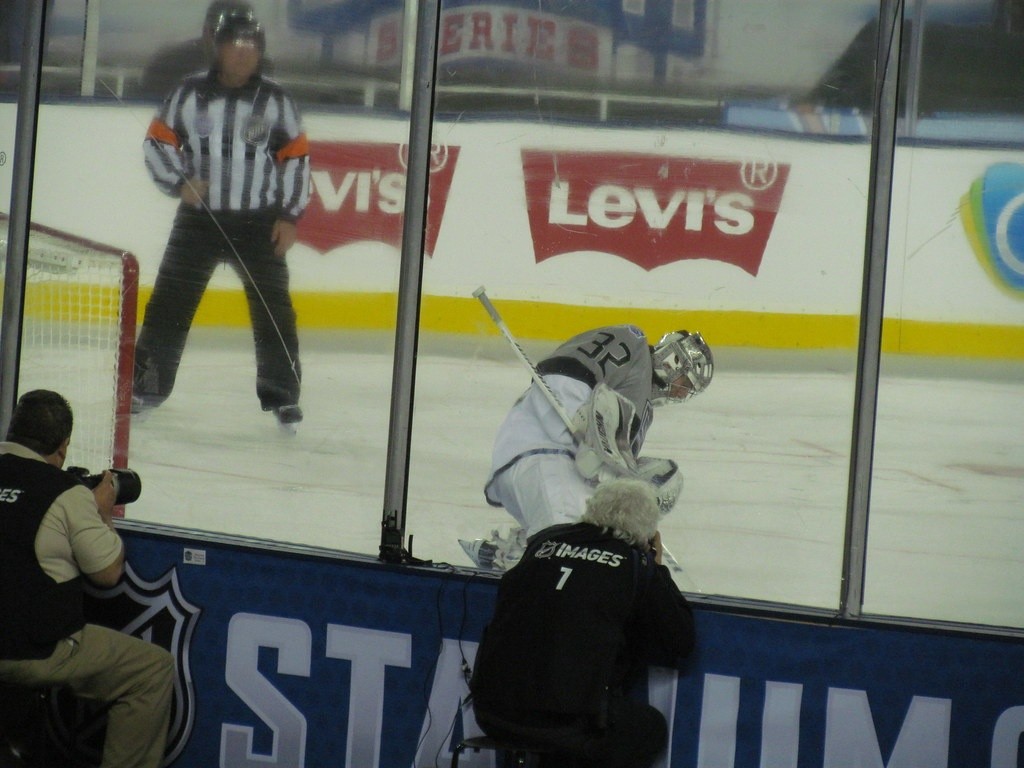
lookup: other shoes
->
[458,537,499,571]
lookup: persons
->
[0,386,176,768]
[456,323,716,573]
[126,0,315,427]
[469,475,696,768]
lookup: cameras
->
[66,466,141,505]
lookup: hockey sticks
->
[471,285,701,594]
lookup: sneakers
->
[112,394,153,423]
[272,403,304,436]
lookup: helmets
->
[203,0,265,63]
[651,329,714,404]
